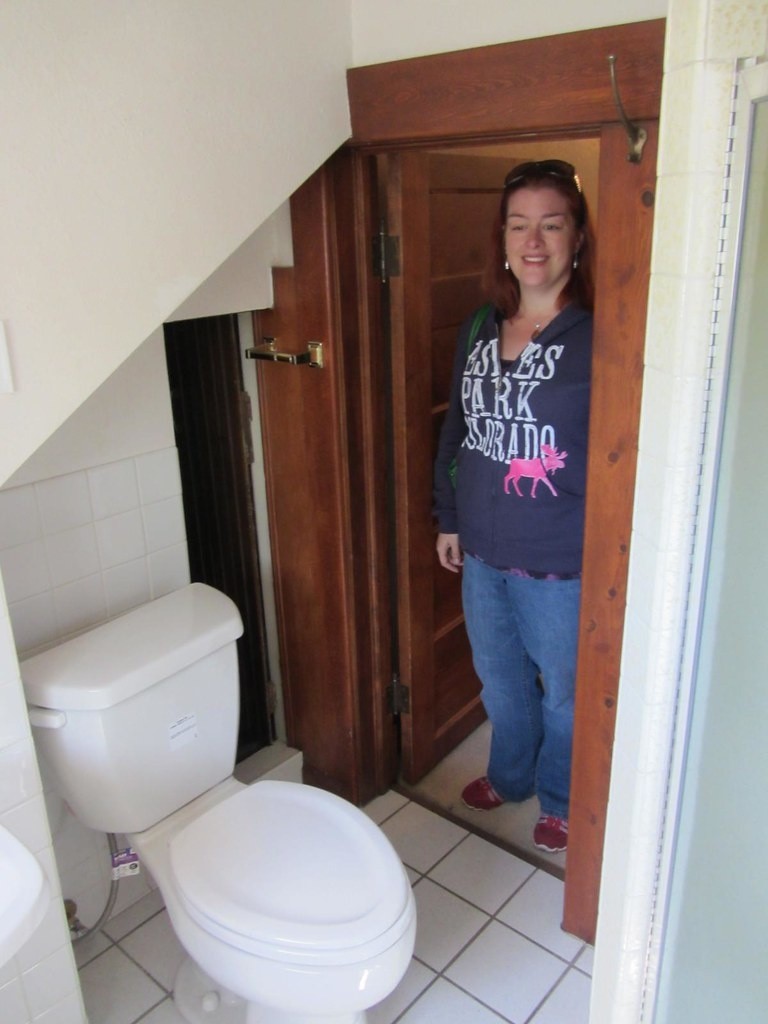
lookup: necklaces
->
[517,301,559,328]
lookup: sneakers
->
[531,816,567,852]
[460,776,504,811]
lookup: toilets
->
[20,581,422,1024]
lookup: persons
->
[434,160,599,851]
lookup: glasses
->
[504,159,581,193]
